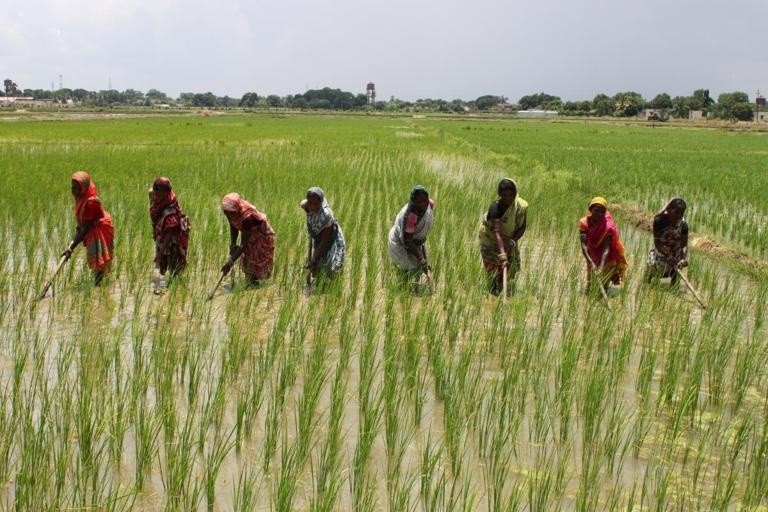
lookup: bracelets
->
[226,257,234,267]
[497,253,508,262]
[310,257,319,265]
[510,238,515,245]
[418,255,426,265]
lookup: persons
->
[67,247,72,252]
[70,170,115,285]
[579,196,628,300]
[478,179,529,297]
[300,187,346,281]
[221,192,275,288]
[148,177,190,276]
[645,198,691,293]
[388,186,435,296]
